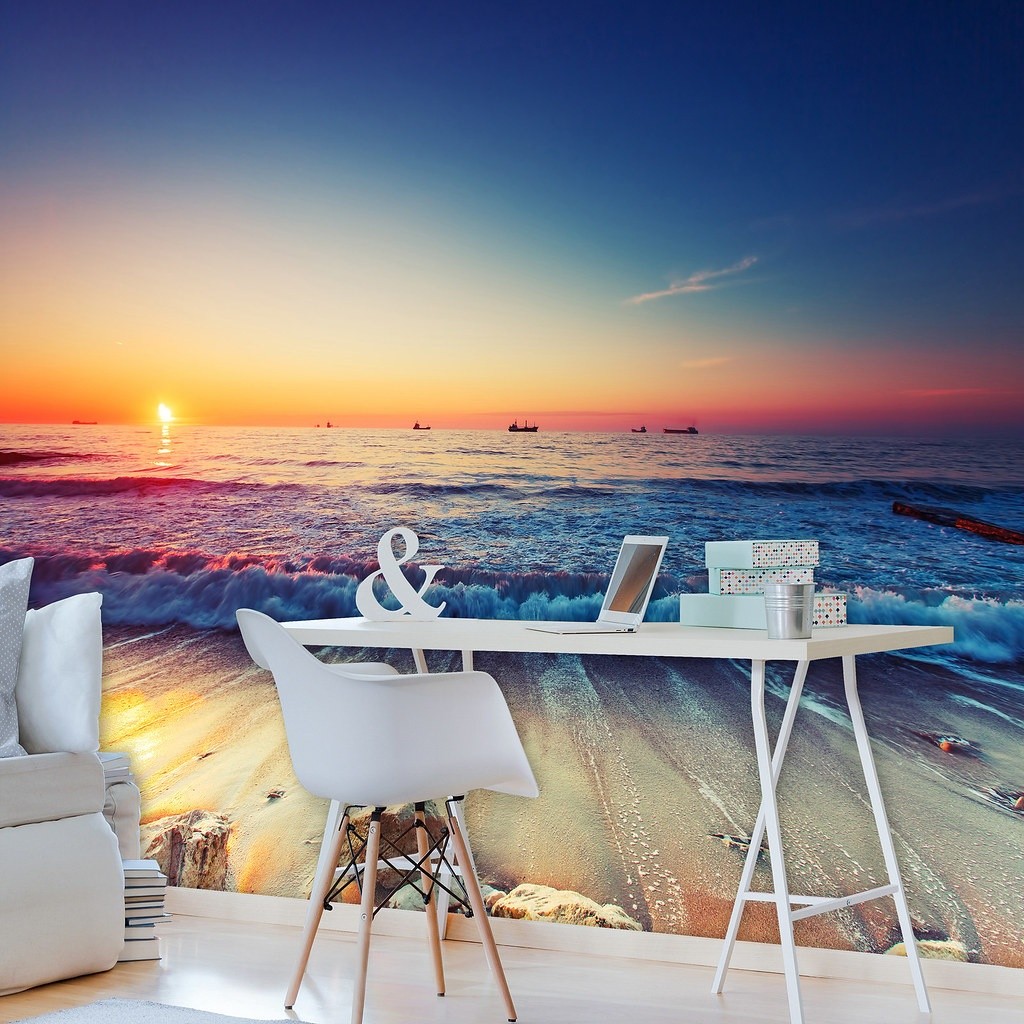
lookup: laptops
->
[526,535,669,634]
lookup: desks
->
[278,616,954,1024]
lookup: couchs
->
[0,752,140,996]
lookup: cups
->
[764,583,816,639]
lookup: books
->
[122,860,173,941]
[96,752,135,789]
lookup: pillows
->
[0,557,33,757]
[15,592,102,754]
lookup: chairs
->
[235,607,539,1024]
